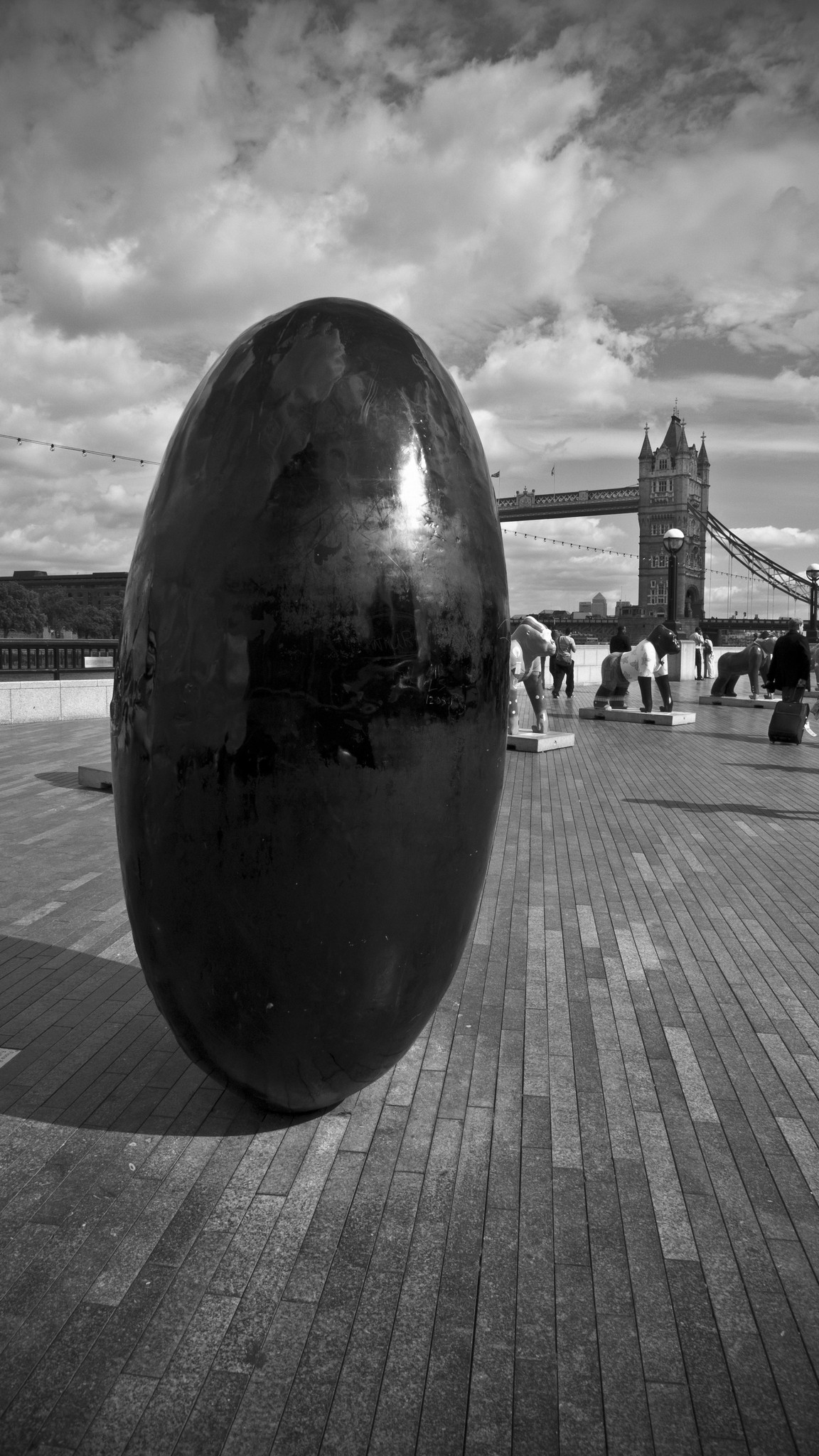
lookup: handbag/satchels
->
[555,653,572,667]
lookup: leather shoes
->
[697,678,706,680]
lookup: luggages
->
[768,684,810,745]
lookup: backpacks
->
[705,639,712,659]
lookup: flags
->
[491,470,500,478]
[551,465,556,476]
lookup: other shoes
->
[568,694,575,698]
[552,693,559,698]
[548,685,555,690]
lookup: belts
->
[696,649,699,650]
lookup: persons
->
[752,633,761,676]
[547,629,562,691]
[764,617,810,703]
[552,627,577,698]
[688,627,707,681]
[609,624,632,695]
[701,634,715,678]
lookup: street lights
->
[662,527,686,639]
[806,562,819,643]
